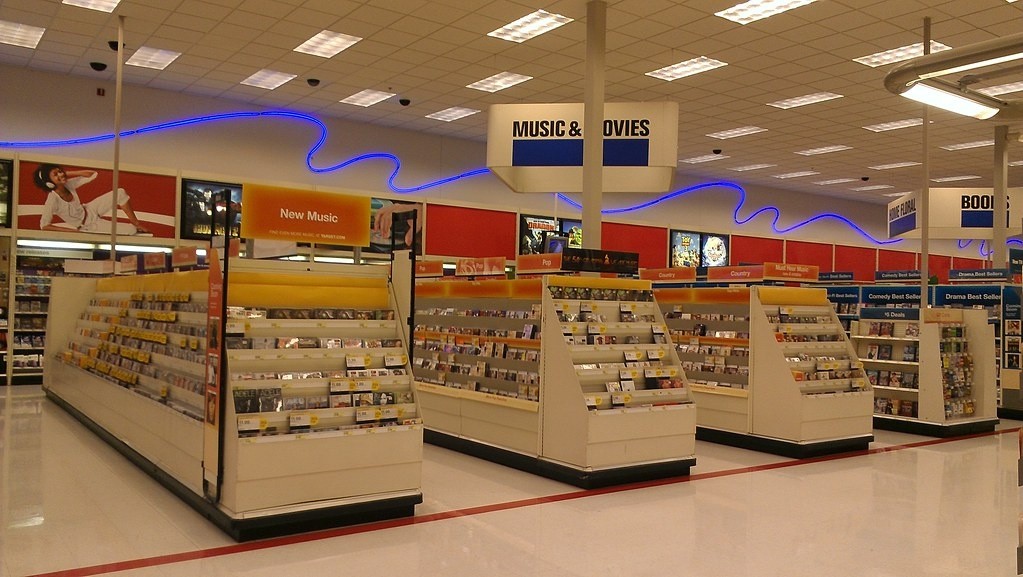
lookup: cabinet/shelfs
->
[43,187,425,544]
[816,282,1023,421]
[0,226,516,387]
[407,247,699,491]
[639,262,876,459]
[851,307,1001,438]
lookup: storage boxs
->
[1,256,976,438]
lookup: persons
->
[34,163,151,236]
